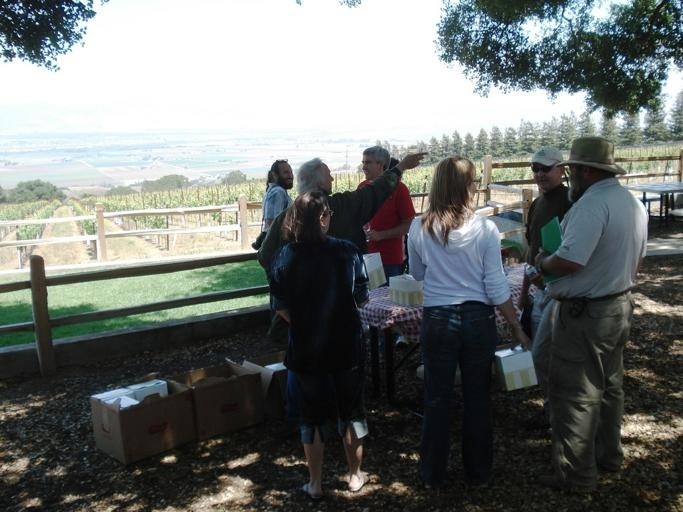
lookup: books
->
[539,216,574,285]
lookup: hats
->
[555,138,626,174]
[530,149,564,167]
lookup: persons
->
[252,159,293,349]
[266,189,372,502]
[407,155,531,490]
[518,146,577,437]
[355,147,415,352]
[533,138,649,493]
[255,150,429,443]
[388,158,403,182]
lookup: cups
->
[363,224,373,242]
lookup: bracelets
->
[391,166,402,178]
[534,252,549,275]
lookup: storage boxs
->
[92,378,194,465]
[177,360,263,443]
[242,350,288,429]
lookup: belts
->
[556,289,630,301]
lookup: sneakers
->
[538,473,597,492]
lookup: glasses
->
[532,166,553,172]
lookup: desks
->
[622,182,683,233]
[360,263,525,409]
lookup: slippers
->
[303,483,322,499]
[349,472,368,491]
